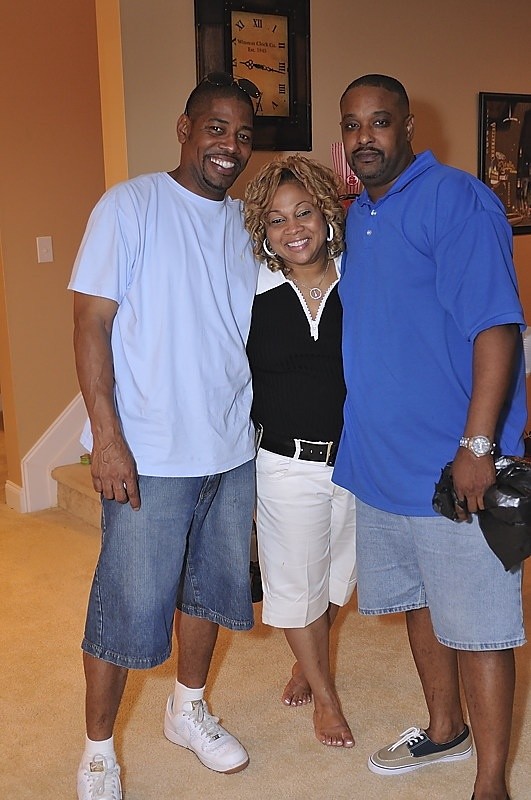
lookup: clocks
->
[192,0,314,153]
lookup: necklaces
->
[284,256,330,300]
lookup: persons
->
[324,74,531,800]
[68,75,263,800]
[239,154,363,746]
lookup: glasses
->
[196,71,262,115]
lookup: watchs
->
[459,435,497,458]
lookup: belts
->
[258,433,337,463]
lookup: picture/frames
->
[477,91,531,235]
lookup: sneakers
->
[163,693,249,774]
[368,723,473,775]
[76,751,123,800]
